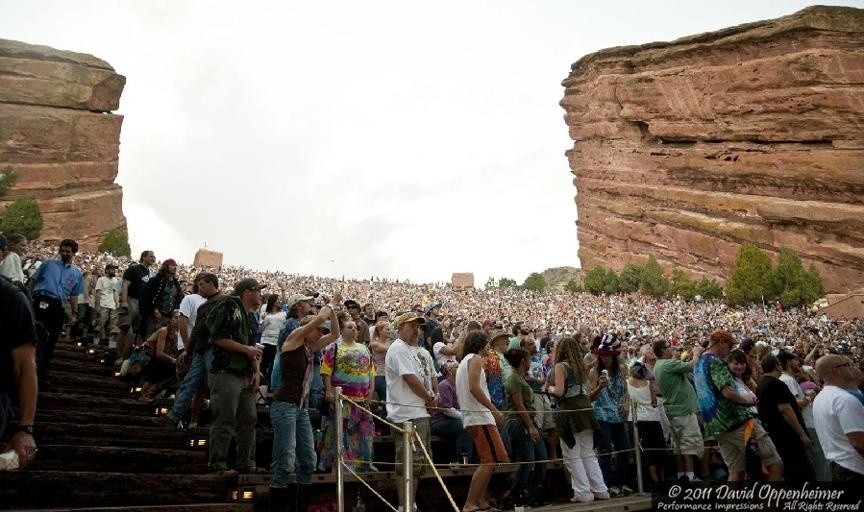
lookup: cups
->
[253,342,264,363]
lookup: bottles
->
[0,449,38,470]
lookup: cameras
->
[256,343,265,360]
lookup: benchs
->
[203,404,656,511]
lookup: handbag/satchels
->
[559,395,591,416]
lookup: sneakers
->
[571,492,610,502]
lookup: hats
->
[236,278,267,294]
[300,315,330,334]
[397,312,425,329]
[344,299,361,309]
[482,321,513,345]
[711,331,738,344]
[426,303,442,313]
[288,289,319,309]
[590,334,621,355]
